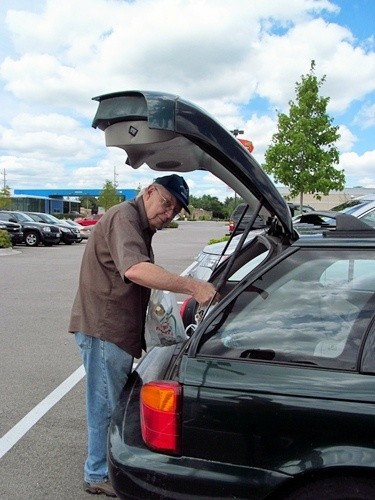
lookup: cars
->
[0,209,103,247]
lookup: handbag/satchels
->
[143,285,189,347]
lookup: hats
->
[155,174,191,215]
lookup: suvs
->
[91,90,375,500]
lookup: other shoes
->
[82,478,116,497]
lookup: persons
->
[67,175,218,497]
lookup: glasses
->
[155,187,181,215]
